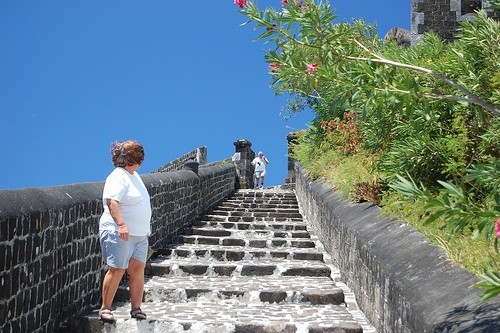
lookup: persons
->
[98,139,153,321]
[250,150,270,189]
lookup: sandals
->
[130,307,147,319]
[99,305,115,324]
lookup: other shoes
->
[261,186,264,189]
[255,186,259,189]
